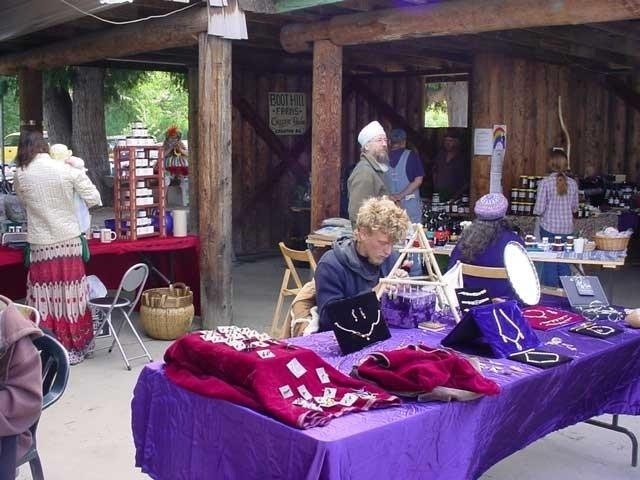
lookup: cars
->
[108,135,157,174]
[1,133,20,164]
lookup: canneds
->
[510,175,543,216]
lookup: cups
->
[573,239,584,254]
[173,209,187,237]
[100,228,117,243]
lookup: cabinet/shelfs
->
[113,145,168,240]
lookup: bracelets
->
[521,297,622,337]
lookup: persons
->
[343,120,400,227]
[532,151,580,289]
[49,142,92,241]
[0,293,41,479]
[429,132,470,201]
[386,126,427,226]
[312,195,412,331]
[445,193,527,310]
[11,129,104,369]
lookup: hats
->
[357,121,386,145]
[50,144,72,162]
[474,192,508,222]
[390,127,407,141]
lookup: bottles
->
[604,183,640,210]
[511,175,543,216]
[422,207,462,246]
[577,191,590,218]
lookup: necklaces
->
[488,306,528,355]
[509,346,560,365]
[332,316,397,345]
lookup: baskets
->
[593,235,630,250]
[139,285,195,340]
